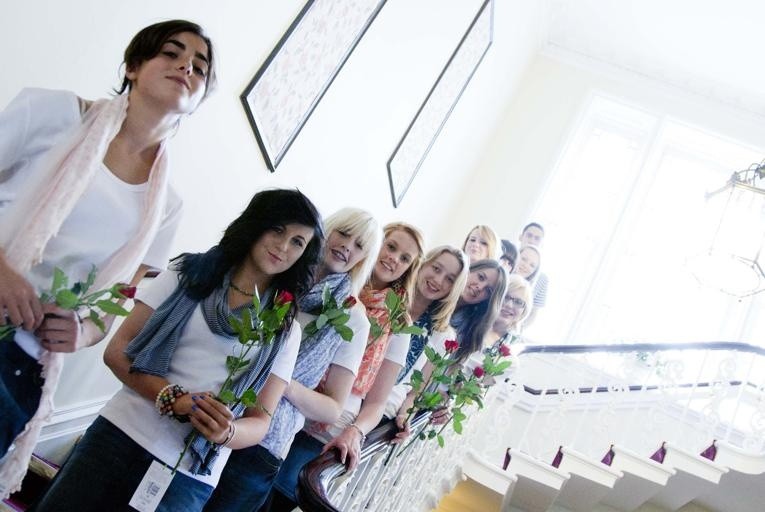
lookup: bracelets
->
[157,384,190,417]
[153,382,178,415]
[345,423,366,453]
[216,420,231,445]
[221,423,236,449]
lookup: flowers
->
[430,352,486,443]
[404,333,460,426]
[364,281,418,342]
[1,265,143,345]
[303,285,355,353]
[162,284,294,476]
[482,340,514,380]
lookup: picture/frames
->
[386,0,497,210]
[238,0,389,175]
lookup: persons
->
[273,221,427,512]
[35,188,328,510]
[203,206,387,512]
[372,221,549,447]
[0,21,214,504]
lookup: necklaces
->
[227,279,269,300]
